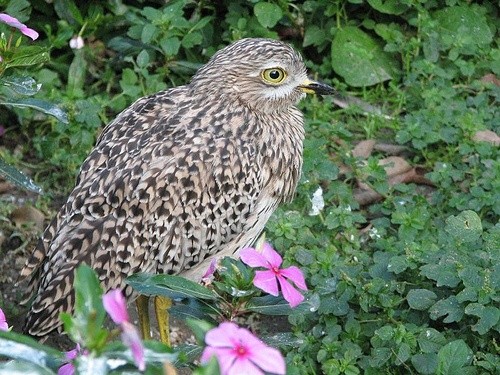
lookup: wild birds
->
[23,37,337,352]
